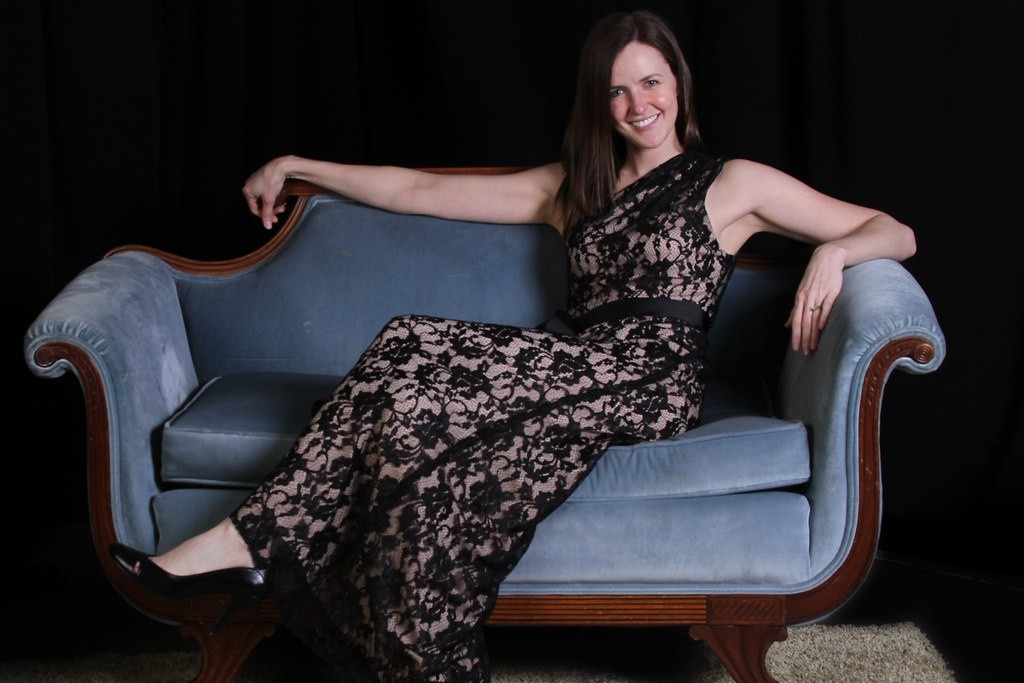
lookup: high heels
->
[109,543,265,635]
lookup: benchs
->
[23,157,949,683]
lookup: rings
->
[810,306,821,311]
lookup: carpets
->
[0,617,961,683]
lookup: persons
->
[105,10,916,683]
